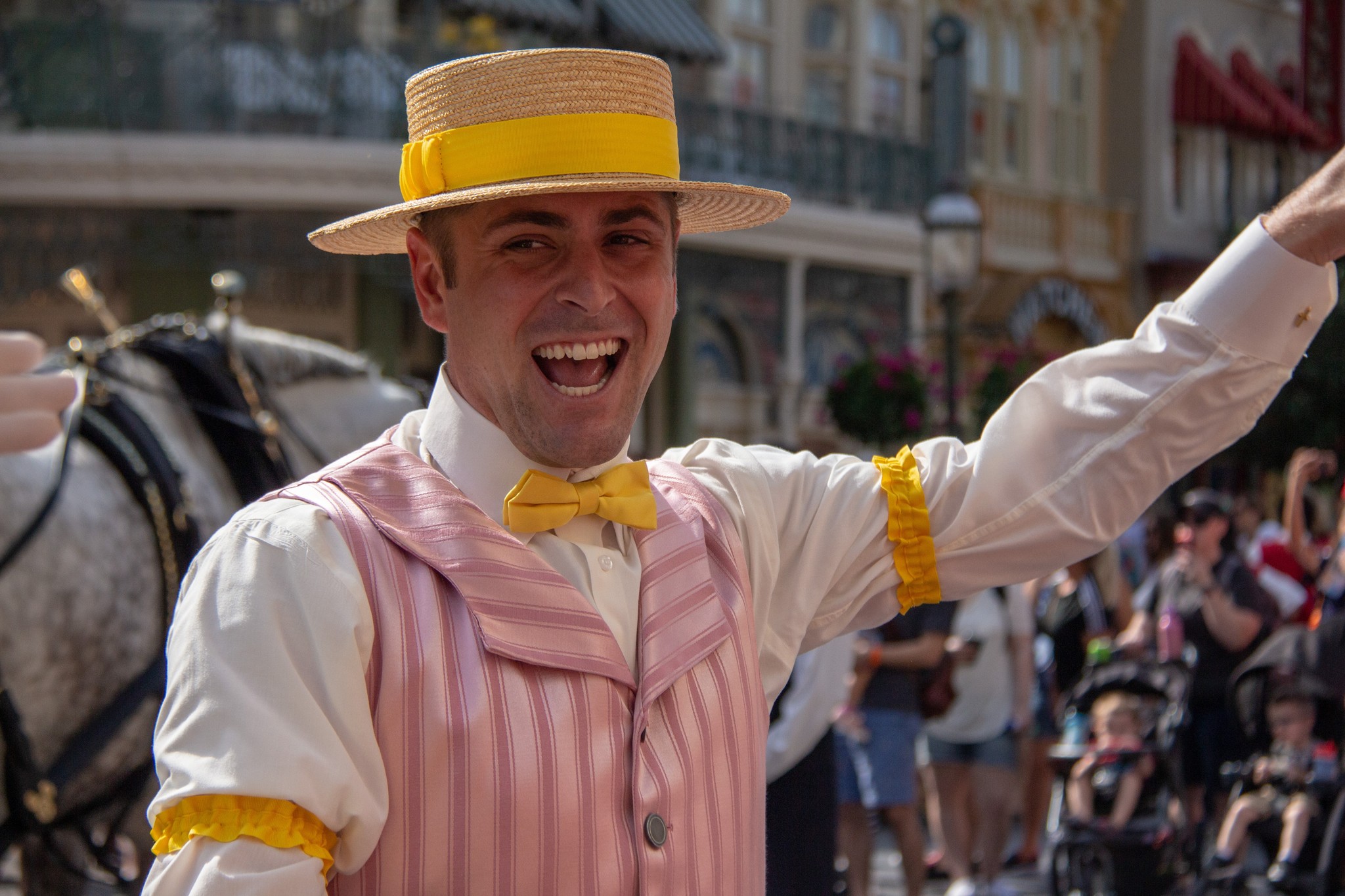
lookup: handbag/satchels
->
[920,656,957,717]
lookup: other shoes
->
[1265,861,1297,888]
[1204,852,1234,876]
[990,876,1017,896]
[999,853,1037,874]
[923,862,950,882]
[833,707,871,746]
[941,876,981,896]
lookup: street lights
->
[919,174,984,437]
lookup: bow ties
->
[502,458,658,533]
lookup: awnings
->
[1229,45,1338,152]
[445,0,727,61]
[1172,38,1276,130]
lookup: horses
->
[1,313,436,896]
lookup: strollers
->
[1033,655,1345,896]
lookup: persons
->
[0,332,78,455]
[138,47,1345,896]
[765,247,1345,896]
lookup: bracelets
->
[869,645,882,670]
[1197,579,1220,597]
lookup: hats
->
[306,47,790,254]
